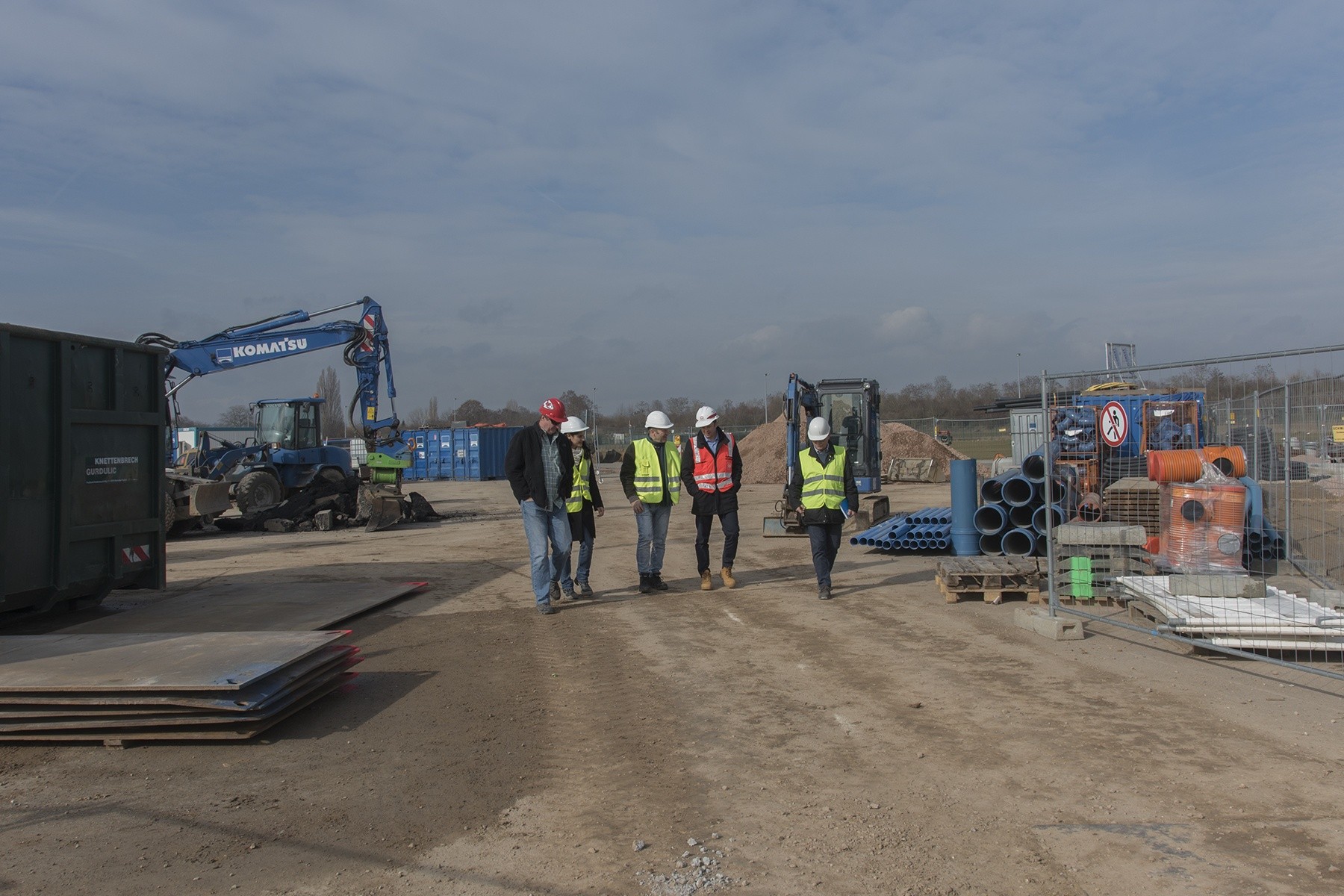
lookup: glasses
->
[550,419,560,425]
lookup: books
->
[839,498,849,519]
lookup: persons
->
[680,406,743,590]
[560,416,604,600]
[620,410,682,593]
[506,398,575,614]
[788,416,859,599]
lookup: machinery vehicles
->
[135,296,444,532]
[759,374,891,534]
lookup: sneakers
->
[564,587,578,599]
[701,571,714,589]
[549,582,561,600]
[639,573,651,592]
[651,574,668,589]
[574,577,592,595]
[537,602,555,614]
[719,566,736,587]
[819,585,830,599]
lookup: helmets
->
[808,416,830,441]
[540,398,568,422]
[696,406,723,427]
[645,410,675,429]
[561,416,589,433]
